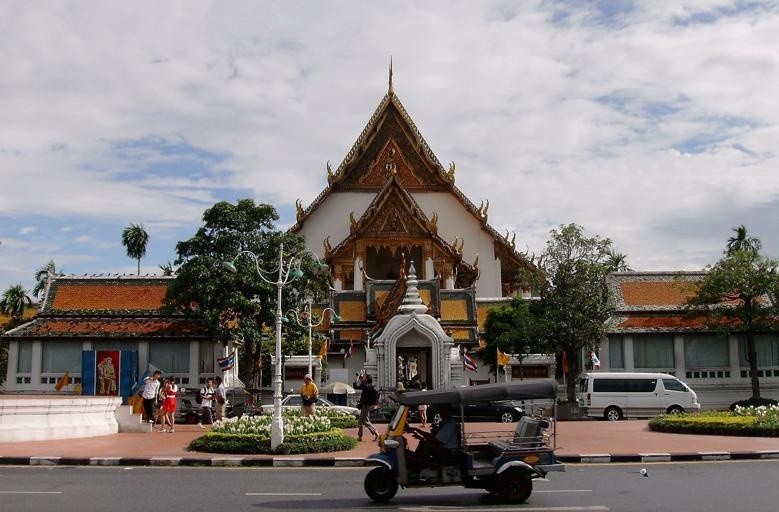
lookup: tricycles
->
[362,377,568,505]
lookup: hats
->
[305,373,312,380]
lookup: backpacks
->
[369,384,380,406]
[195,386,206,405]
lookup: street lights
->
[221,239,344,454]
[511,345,530,417]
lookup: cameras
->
[356,372,363,379]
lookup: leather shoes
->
[157,428,176,433]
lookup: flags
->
[217,352,234,372]
[496,347,514,366]
[563,351,568,373]
[319,340,327,358]
[344,342,352,360]
[464,351,478,373]
[55,371,68,392]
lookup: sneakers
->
[197,423,206,429]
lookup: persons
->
[213,376,227,420]
[300,373,318,416]
[417,381,428,428]
[352,373,380,441]
[104,359,117,394]
[590,350,600,370]
[143,370,163,426]
[155,379,172,426]
[407,405,461,466]
[198,378,216,428]
[157,377,179,433]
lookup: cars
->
[411,398,528,423]
[255,393,361,420]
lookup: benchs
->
[488,416,543,464]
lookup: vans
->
[578,371,702,421]
